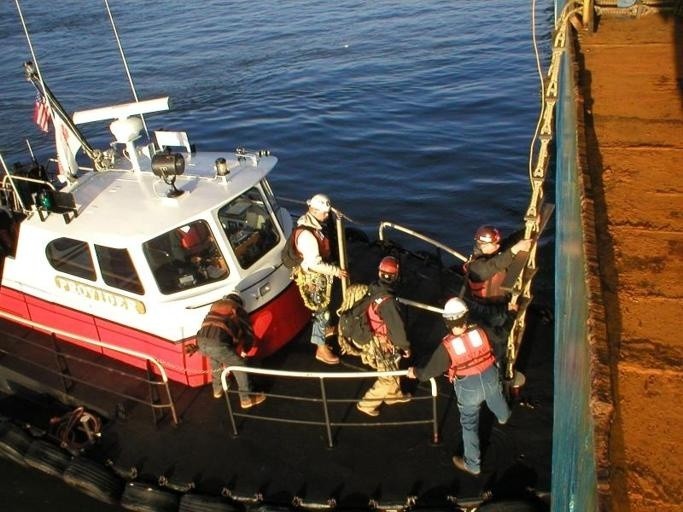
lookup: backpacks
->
[339,284,394,345]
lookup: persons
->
[170,221,216,265]
[407,296,512,476]
[11,158,56,212]
[196,289,266,409]
[463,223,535,382]
[356,255,412,417]
[291,192,350,366]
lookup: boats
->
[0,0,553,511]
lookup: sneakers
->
[384,392,413,405]
[453,458,480,474]
[316,344,340,365]
[240,392,267,408]
[357,402,379,416]
[325,324,339,337]
[213,378,232,398]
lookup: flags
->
[29,78,81,183]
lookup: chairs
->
[177,224,209,251]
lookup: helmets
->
[442,297,468,318]
[222,290,246,308]
[307,194,331,212]
[378,256,400,274]
[474,225,501,243]
